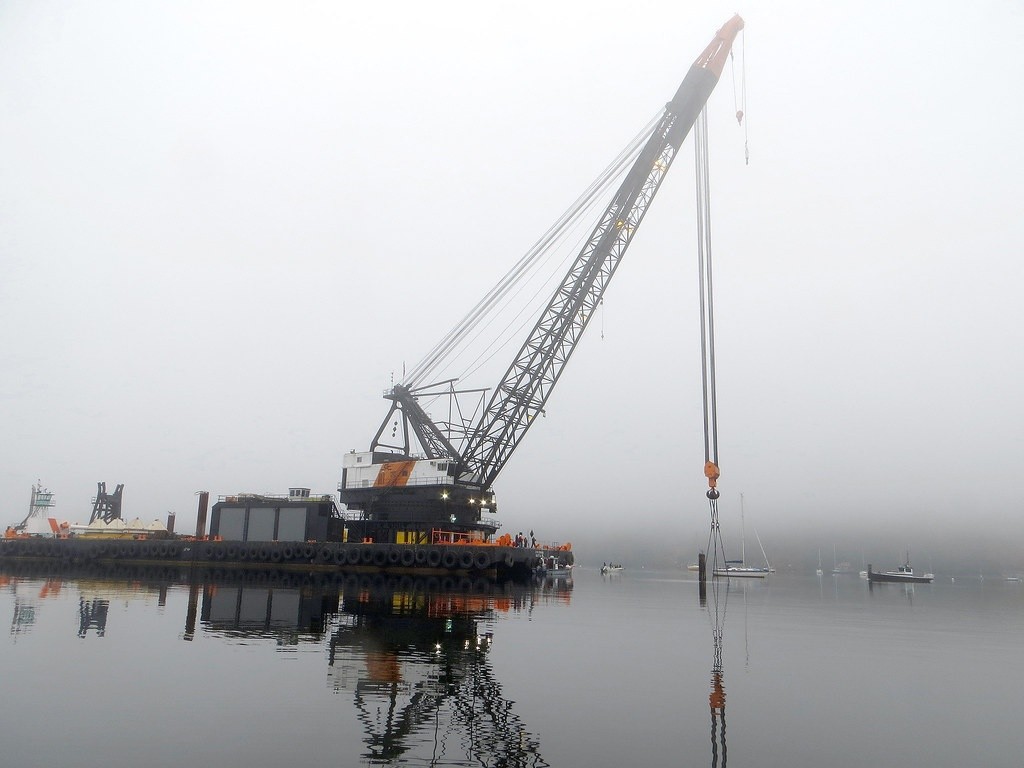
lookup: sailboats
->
[816,547,823,576]
[871,552,935,583]
[859,546,869,578]
[831,544,839,577]
[713,492,774,582]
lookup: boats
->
[601,562,624,574]
[1002,578,1020,583]
[546,565,573,578]
[976,575,984,585]
[688,565,699,571]
[951,576,960,583]
[837,561,850,573]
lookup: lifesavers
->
[373,548,388,565]
[388,549,401,564]
[441,551,459,568]
[401,548,415,566]
[474,551,490,570]
[319,547,333,562]
[505,553,516,567]
[525,558,535,570]
[334,548,347,566]
[361,546,373,563]
[427,550,442,567]
[347,547,361,565]
[415,549,427,564]
[0,540,316,564]
[459,550,474,569]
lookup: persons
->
[604,561,621,568]
[524,537,527,548]
[530,537,536,548]
